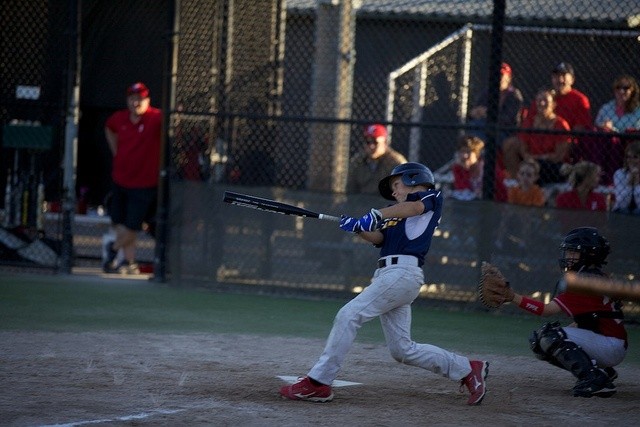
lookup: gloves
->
[338,212,363,234]
[357,207,385,232]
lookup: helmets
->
[378,162,435,201]
[558,233,610,274]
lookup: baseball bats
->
[221,191,341,222]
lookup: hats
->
[127,82,149,98]
[363,123,388,139]
[499,62,513,80]
[550,61,575,77]
[457,135,485,158]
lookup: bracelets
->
[517,297,545,317]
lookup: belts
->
[376,256,426,268]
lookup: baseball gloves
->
[479,260,514,310]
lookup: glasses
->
[614,85,632,90]
[365,141,378,144]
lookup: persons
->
[518,87,572,186]
[435,136,486,203]
[471,63,523,153]
[419,71,462,170]
[173,93,212,184]
[431,135,508,203]
[590,74,640,170]
[230,100,278,190]
[523,60,593,140]
[505,155,542,207]
[487,226,629,398]
[608,142,640,214]
[104,81,162,278]
[277,160,490,407]
[346,124,410,201]
[556,161,611,211]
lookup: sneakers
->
[117,259,139,274]
[569,367,617,395]
[597,365,618,381]
[105,241,118,267]
[459,359,489,405]
[278,375,334,402]
